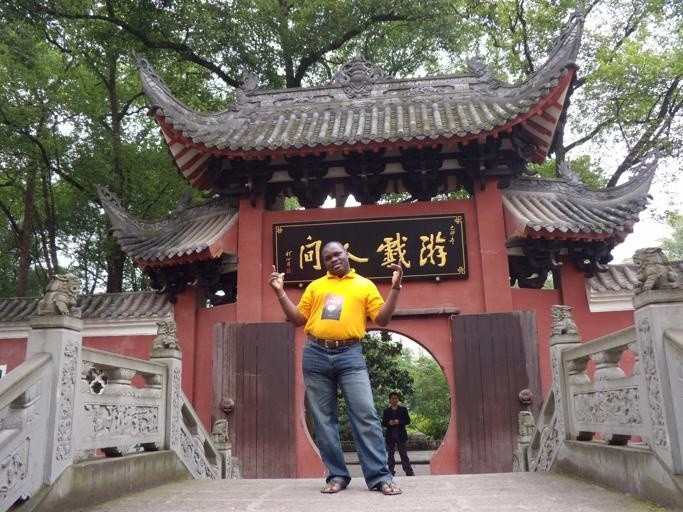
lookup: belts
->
[306,331,359,347]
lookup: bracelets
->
[391,285,402,290]
[276,292,285,299]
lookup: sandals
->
[321,476,346,493]
[376,479,402,495]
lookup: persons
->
[267,238,408,494]
[382,392,415,476]
